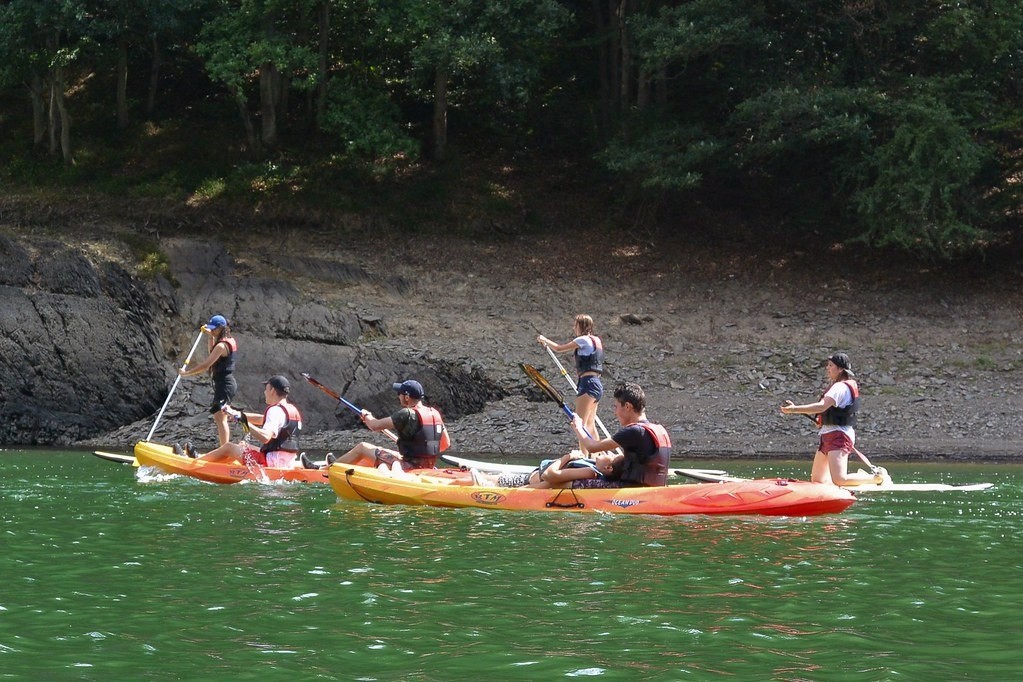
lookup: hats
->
[828,354,854,377]
[207,315,226,329]
[261,375,290,394]
[393,380,424,399]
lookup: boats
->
[135,440,472,486]
[324,458,858,516]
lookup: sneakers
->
[326,453,336,467]
[300,452,320,469]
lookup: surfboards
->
[441,455,728,481]
[675,469,995,494]
[440,452,726,478]
[92,450,137,467]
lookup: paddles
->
[301,370,465,469]
[132,332,203,468]
[519,362,609,454]
[760,381,882,475]
[219,398,250,435]
[541,339,624,455]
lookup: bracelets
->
[208,337,213,339]
[364,412,371,421]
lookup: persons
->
[173,375,302,468]
[521,383,671,490]
[180,315,237,445]
[378,450,626,488]
[782,354,892,486]
[300,379,450,470]
[537,315,603,455]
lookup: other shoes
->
[173,443,184,456]
[186,442,199,458]
[874,466,893,485]
[857,468,869,474]
[472,469,494,486]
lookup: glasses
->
[397,391,407,396]
